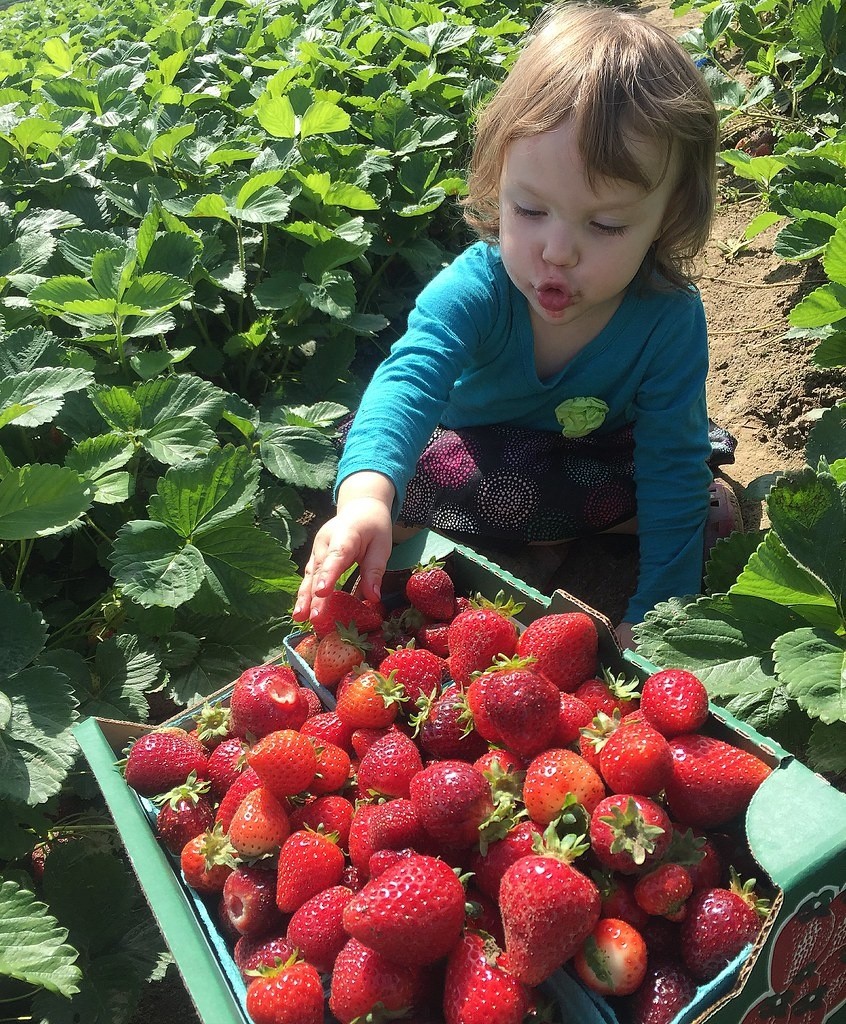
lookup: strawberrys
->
[122,556,772,1024]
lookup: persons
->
[292,3,744,652]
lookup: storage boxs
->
[71,528,846,1024]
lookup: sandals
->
[704,478,742,583]
